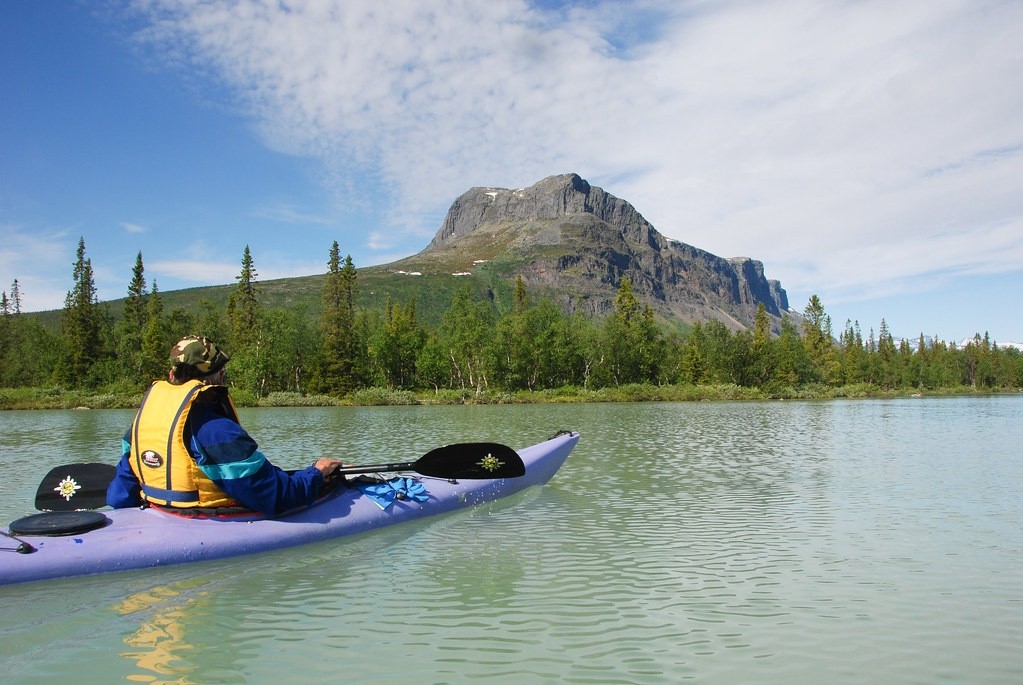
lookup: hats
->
[168,335,231,376]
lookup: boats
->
[0,430,580,585]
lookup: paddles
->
[34,442,527,512]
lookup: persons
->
[105,334,345,517]
